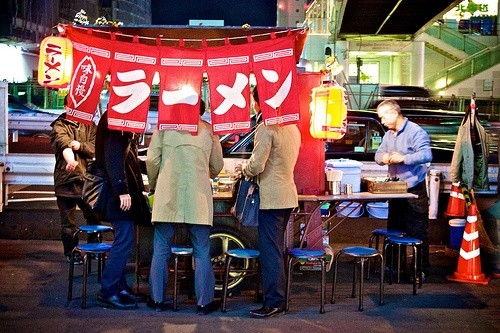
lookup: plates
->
[218,178,237,184]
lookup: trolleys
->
[134,194,294,296]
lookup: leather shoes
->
[146,301,164,311]
[121,287,146,301]
[249,306,283,317]
[198,300,222,314]
[97,294,138,309]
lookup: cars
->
[4,94,60,139]
[216,84,500,172]
[102,88,159,135]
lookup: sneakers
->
[86,252,108,260]
[67,254,83,265]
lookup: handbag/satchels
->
[230,171,259,226]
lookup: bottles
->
[212,175,219,193]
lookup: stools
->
[383,236,423,297]
[369,228,406,287]
[220,249,260,313]
[284,247,328,315]
[330,245,385,312]
[67,242,113,309]
[73,224,115,275]
[170,245,193,312]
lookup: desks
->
[137,190,419,284]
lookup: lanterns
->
[38,36,73,88]
[309,81,348,142]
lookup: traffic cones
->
[445,204,492,285]
[444,177,465,218]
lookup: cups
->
[344,184,352,194]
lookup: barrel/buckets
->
[366,202,388,219]
[320,202,331,217]
[335,202,364,218]
[449,219,466,248]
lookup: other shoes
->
[376,265,400,274]
[411,270,428,283]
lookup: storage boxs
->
[449,219,466,250]
[325,159,363,193]
[362,177,408,194]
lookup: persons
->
[375,99,433,284]
[95,110,144,310]
[146,98,224,313]
[235,84,301,318]
[49,93,107,265]
